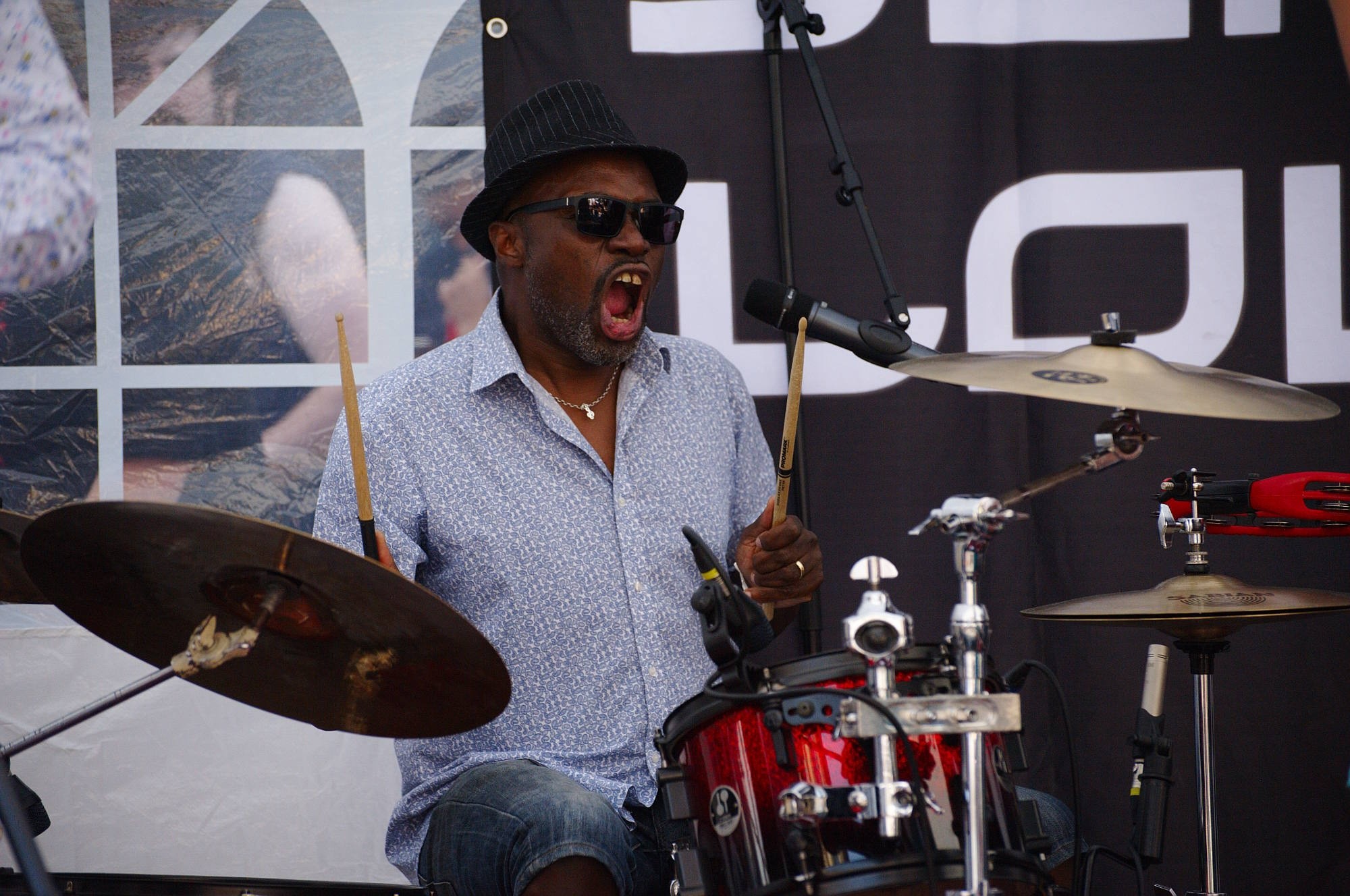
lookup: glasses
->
[500,191,684,247]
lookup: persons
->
[0,0,497,540]
[313,81,826,896]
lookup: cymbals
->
[1148,468,1350,540]
[22,497,517,743]
[1017,570,1350,639]
[885,343,1343,424]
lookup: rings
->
[796,561,805,580]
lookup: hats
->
[460,78,690,262]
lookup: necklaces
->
[549,361,623,420]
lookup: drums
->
[656,645,1056,896]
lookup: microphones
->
[691,537,775,654]
[8,773,50,836]
[743,279,943,367]
[1130,644,1171,825]
